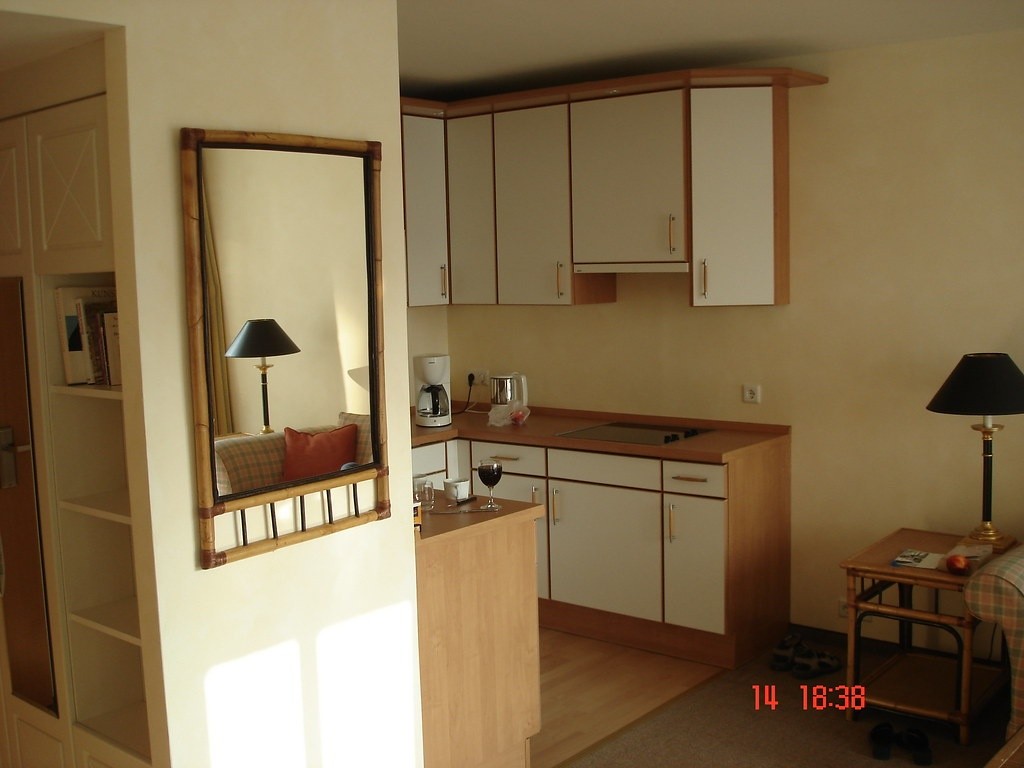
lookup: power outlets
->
[742,382,761,402]
[465,369,489,390]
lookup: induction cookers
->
[557,422,716,446]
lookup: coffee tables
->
[840,524,996,740]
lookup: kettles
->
[488,372,529,410]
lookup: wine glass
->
[478,459,502,510]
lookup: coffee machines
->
[414,355,451,427]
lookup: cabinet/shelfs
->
[665,449,792,634]
[449,103,572,305]
[405,108,446,305]
[572,81,795,308]
[412,442,447,491]
[469,443,662,622]
[29,93,169,768]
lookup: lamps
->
[221,317,301,432]
[924,352,1024,549]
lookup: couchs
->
[215,406,369,494]
[961,546,1024,740]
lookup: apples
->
[510,409,524,424]
[947,555,970,575]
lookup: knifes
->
[429,508,499,514]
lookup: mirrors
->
[178,126,391,572]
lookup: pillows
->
[281,423,357,481]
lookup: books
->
[937,544,993,576]
[893,550,942,569]
[55,286,122,386]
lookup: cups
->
[413,478,436,511]
[443,477,471,500]
